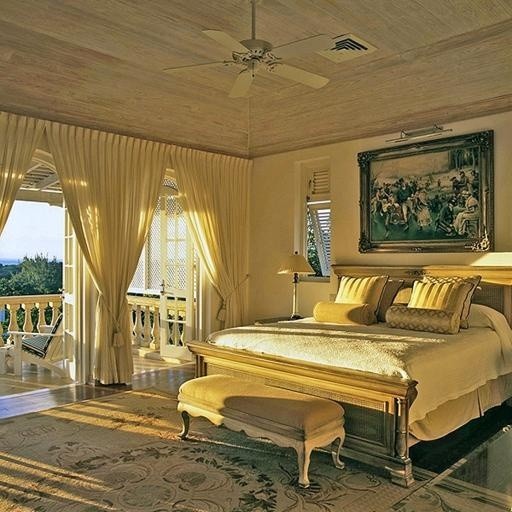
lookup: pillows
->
[312,273,484,336]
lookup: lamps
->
[271,251,316,321]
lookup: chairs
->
[7,313,69,378]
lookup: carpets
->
[0,385,511,511]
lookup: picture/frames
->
[357,129,494,255]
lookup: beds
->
[185,263,512,489]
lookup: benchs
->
[176,373,347,489]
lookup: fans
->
[160,1,331,99]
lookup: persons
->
[375,171,479,237]
